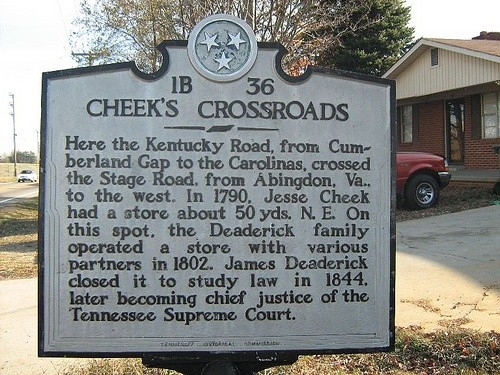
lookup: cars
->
[18,169,38,182]
[396,152,452,210]
